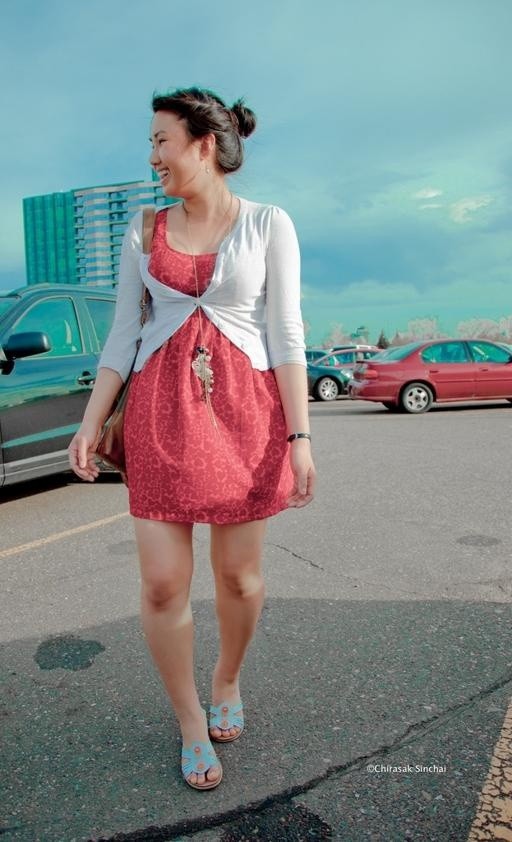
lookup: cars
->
[303,341,511,411]
[1,285,122,488]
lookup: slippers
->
[209,683,244,743]
[180,737,224,790]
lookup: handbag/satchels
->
[94,336,140,485]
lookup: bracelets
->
[284,433,313,441]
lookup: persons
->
[66,81,318,792]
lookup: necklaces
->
[182,190,239,417]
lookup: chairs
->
[42,315,78,356]
[448,346,464,363]
